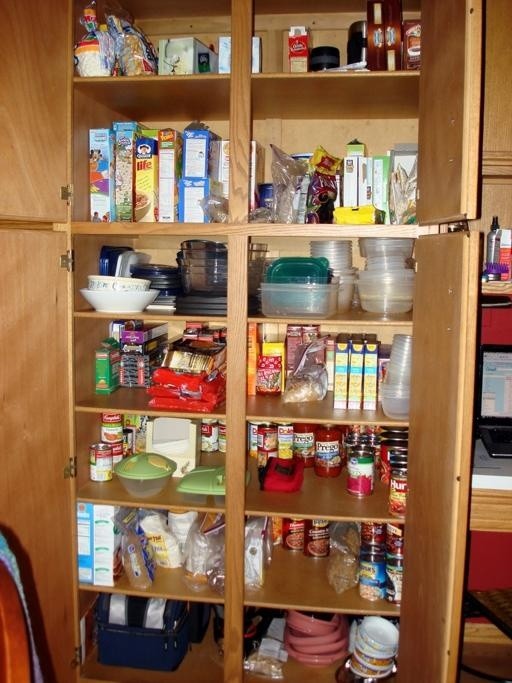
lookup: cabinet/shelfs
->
[0,0,485,683]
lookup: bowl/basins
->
[114,451,177,498]
[79,274,160,313]
[179,240,270,294]
[350,615,399,679]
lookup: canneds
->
[87,411,227,484]
[246,422,409,607]
[289,153,314,224]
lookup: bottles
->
[486,216,501,281]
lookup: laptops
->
[478,343,511,458]
